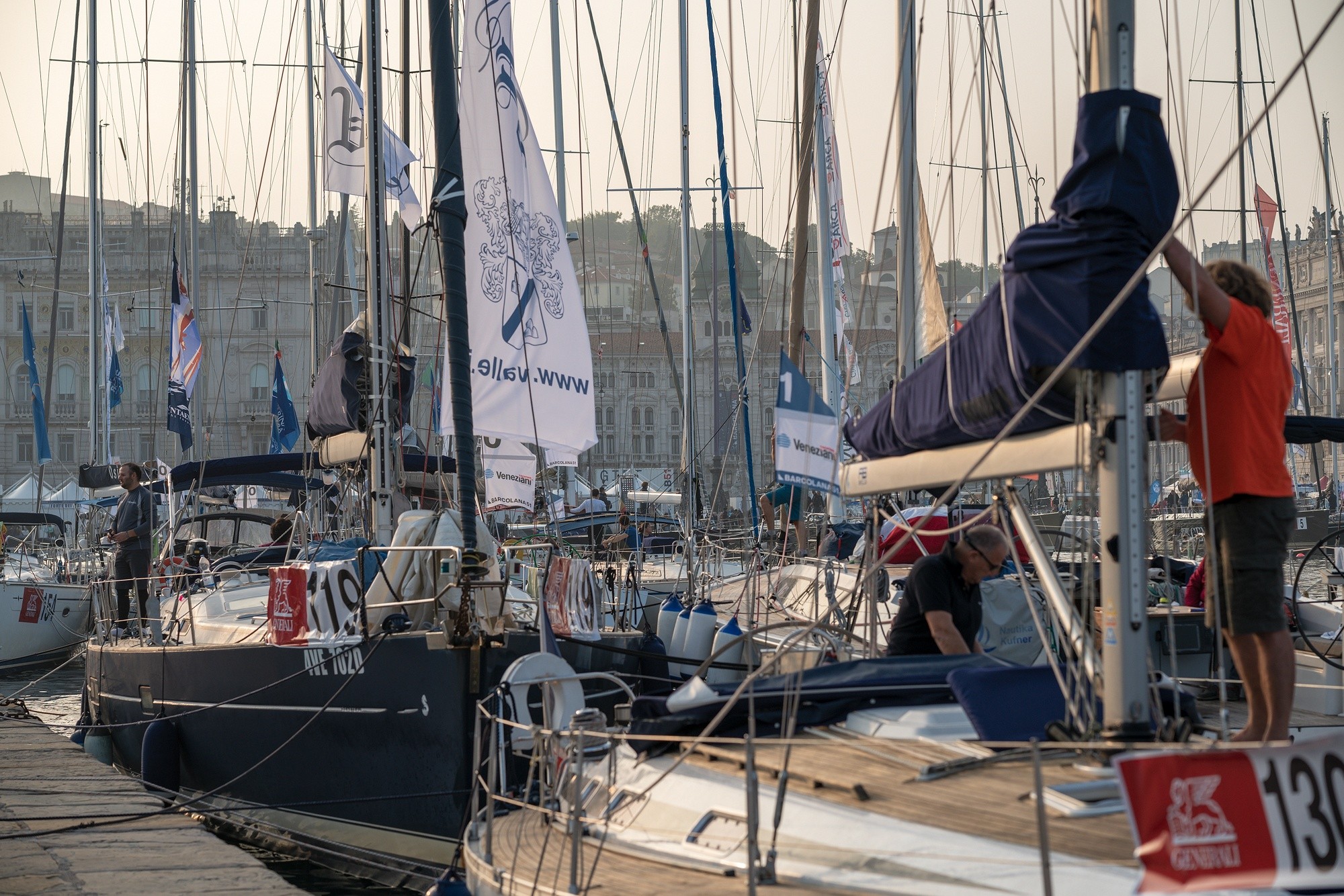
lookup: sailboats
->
[0,0,1343,896]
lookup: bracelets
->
[126,531,130,539]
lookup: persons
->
[503,513,530,524]
[886,526,1010,658]
[1285,210,1344,248]
[107,464,159,637]
[1316,490,1337,513]
[810,490,825,513]
[601,514,656,569]
[753,420,809,562]
[569,487,613,551]
[662,507,752,531]
[1151,236,1300,747]
[640,481,649,515]
[258,519,304,549]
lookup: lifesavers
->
[158,557,188,588]
[499,652,587,749]
[502,539,524,562]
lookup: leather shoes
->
[754,531,777,542]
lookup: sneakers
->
[112,627,124,638]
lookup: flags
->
[102,259,126,463]
[323,37,423,232]
[168,234,203,451]
[440,3,597,517]
[268,339,302,456]
[775,349,845,499]
[1254,183,1311,456]
[817,29,862,523]
[22,302,52,467]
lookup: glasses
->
[981,554,999,570]
